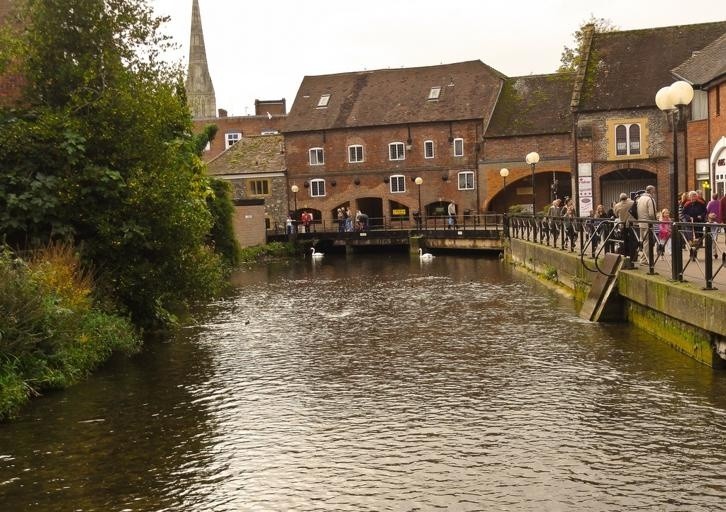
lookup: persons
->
[541,184,726,266]
[337,204,371,233]
[301,210,311,232]
[447,200,460,230]
[285,216,293,234]
[412,207,423,230]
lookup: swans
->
[310,246,325,256]
[418,248,433,258]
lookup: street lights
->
[526,149,539,231]
[499,166,509,230]
[290,181,299,232]
[655,78,694,284]
[413,176,424,230]
